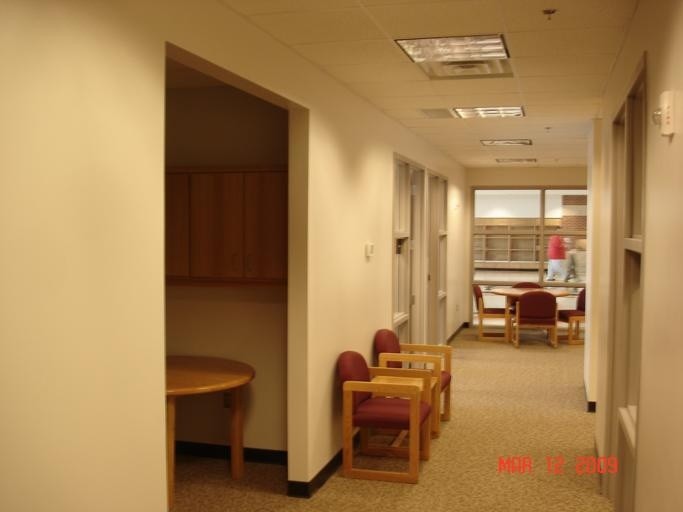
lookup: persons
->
[545,225,571,290]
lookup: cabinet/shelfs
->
[166,167,288,285]
[472,225,558,261]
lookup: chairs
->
[337,350,432,483]
[472,282,585,347]
[374,329,452,437]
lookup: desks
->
[166,355,255,512]
[371,376,438,394]
